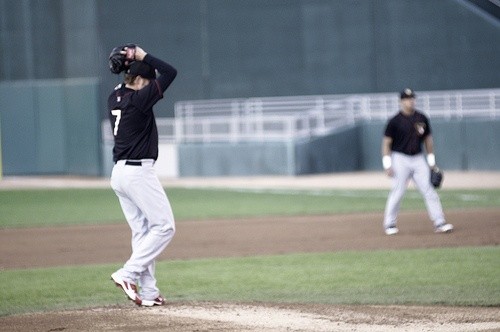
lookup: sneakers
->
[434,224,454,233]
[111,268,143,306]
[140,295,167,307]
[384,225,399,236]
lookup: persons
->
[107,44,177,307]
[381,87,456,236]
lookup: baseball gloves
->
[430,170,443,187]
[108,44,137,74]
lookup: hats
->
[124,59,158,80]
[401,87,415,98]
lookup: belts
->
[126,162,142,166]
